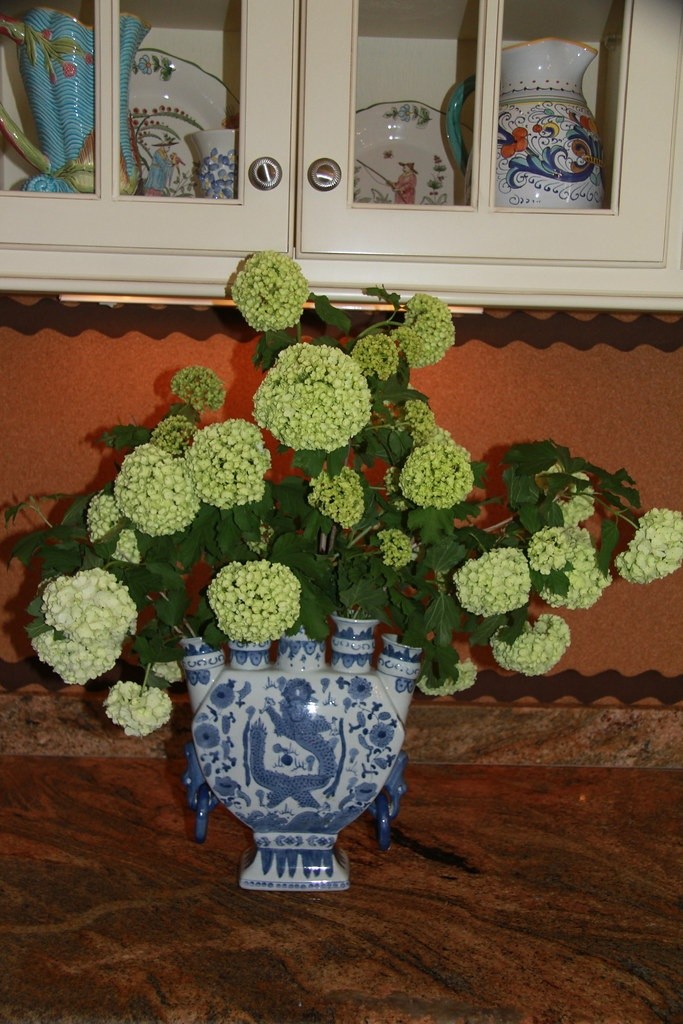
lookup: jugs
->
[446,35,605,210]
[0,6,155,196]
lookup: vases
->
[174,610,424,890]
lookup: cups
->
[190,129,238,199]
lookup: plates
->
[127,48,241,199]
[352,101,473,206]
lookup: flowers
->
[5,250,681,737]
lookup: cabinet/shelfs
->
[3,2,680,312]
